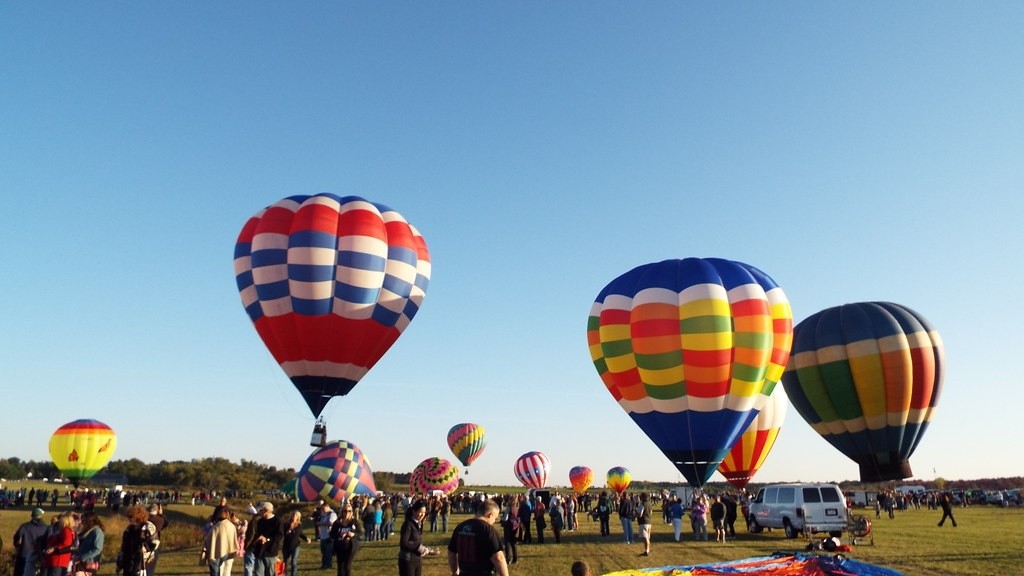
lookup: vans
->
[748,483,848,540]
[895,486,927,496]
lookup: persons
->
[282,510,311,576]
[710,495,727,542]
[637,492,652,556]
[0,482,1024,576]
[447,498,508,576]
[398,500,438,576]
[669,498,685,541]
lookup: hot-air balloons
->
[716,379,789,493]
[606,467,631,498]
[569,466,593,497]
[513,450,552,490]
[447,422,487,475]
[233,191,431,450]
[780,301,946,487]
[410,456,460,497]
[49,418,117,489]
[295,439,378,505]
[586,254,793,513]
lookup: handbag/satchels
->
[503,507,510,521]
[66,556,92,576]
[86,551,99,571]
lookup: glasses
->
[260,508,264,512]
[343,509,352,513]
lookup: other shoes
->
[364,538,367,541]
[378,538,381,541]
[383,538,386,541]
[642,548,650,556]
[386,537,388,541]
[369,539,371,542]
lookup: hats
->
[31,507,44,516]
